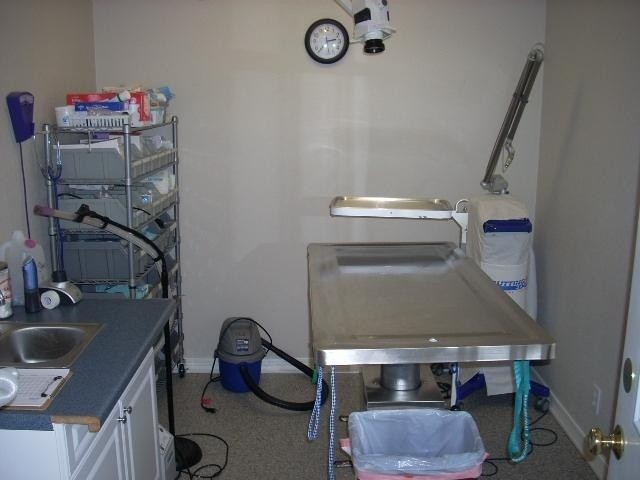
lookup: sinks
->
[1,321,105,369]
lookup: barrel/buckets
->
[478,251,530,336]
[0,231,50,307]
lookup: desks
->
[308,242,554,466]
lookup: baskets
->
[69,110,134,130]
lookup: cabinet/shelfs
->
[1,349,162,479]
[44,116,184,377]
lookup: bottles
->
[0,261,13,321]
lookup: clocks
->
[304,18,349,65]
